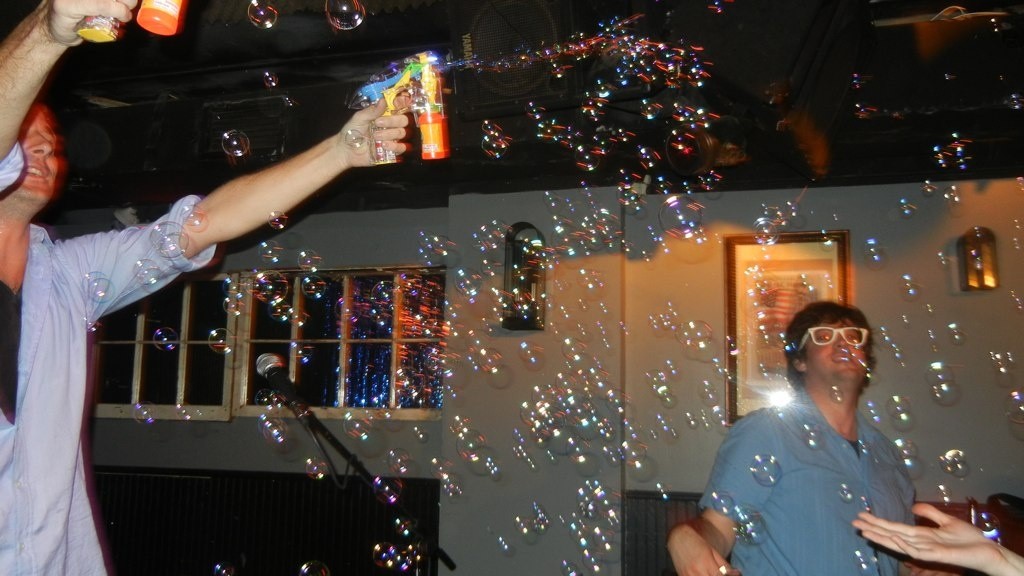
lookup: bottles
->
[418,111,451,161]
[134,0,188,37]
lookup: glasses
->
[798,326,868,347]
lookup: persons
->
[851,502,1024,576]
[668,301,959,576]
[1,0,414,576]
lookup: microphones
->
[256,353,309,426]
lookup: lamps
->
[961,227,997,295]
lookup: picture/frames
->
[722,229,852,423]
